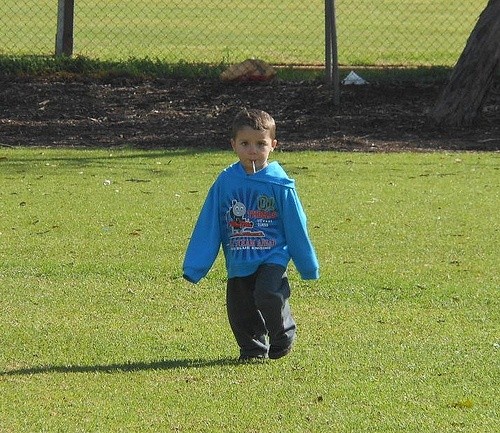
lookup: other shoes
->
[268,345,292,359]
[239,353,266,361]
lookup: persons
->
[184,106,320,364]
